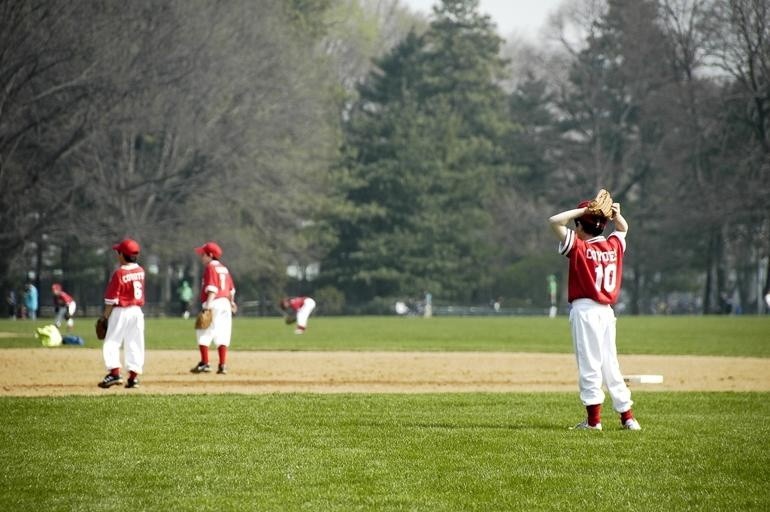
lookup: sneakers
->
[98,373,124,389]
[125,378,140,389]
[189,362,212,374]
[622,417,642,431]
[216,367,227,375]
[568,418,603,431]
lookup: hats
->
[111,238,140,257]
[576,200,608,225]
[193,241,222,257]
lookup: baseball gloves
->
[587,189,613,221]
[195,309,212,329]
[96,319,108,339]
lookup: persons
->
[545,273,559,319]
[423,289,433,317]
[50,282,77,335]
[190,240,237,374]
[178,279,192,319]
[548,201,642,432]
[97,240,143,390]
[280,295,316,335]
[1,282,39,322]
